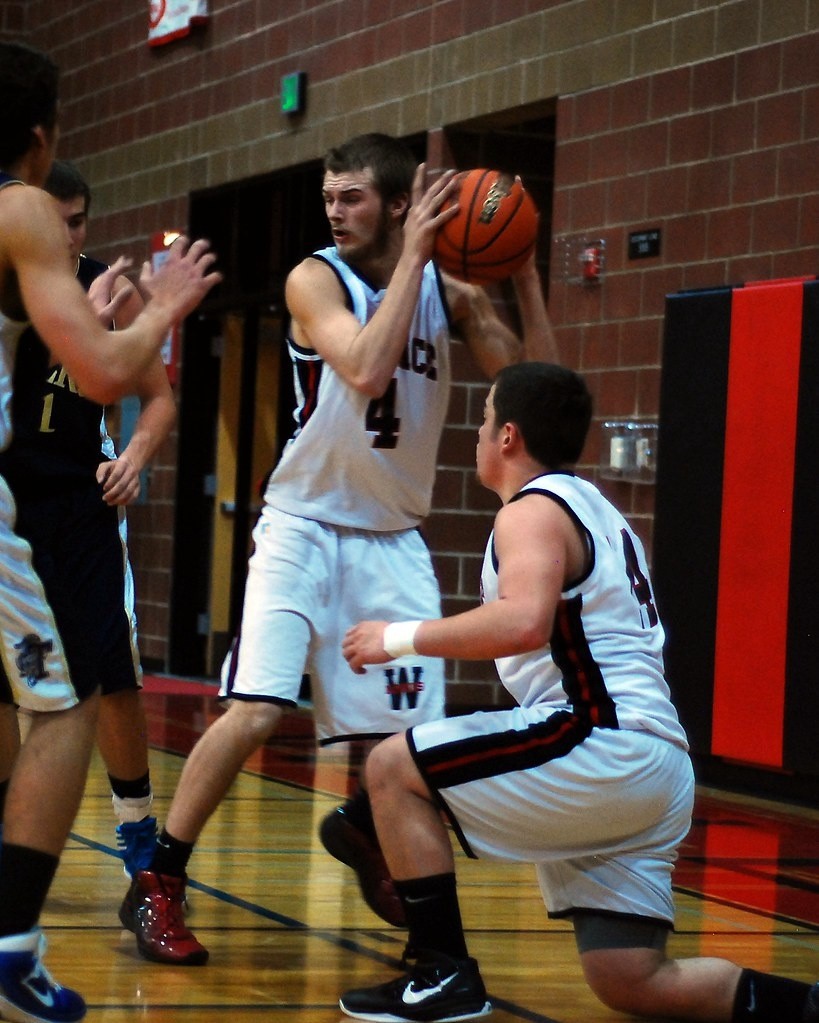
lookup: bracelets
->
[383,620,424,659]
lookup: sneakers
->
[0,926,86,1023]
[117,869,208,965]
[116,816,159,878]
[338,957,491,1023]
[321,805,407,926]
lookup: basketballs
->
[432,167,538,284]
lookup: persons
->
[118,131,561,965]
[41,161,210,911]
[340,361,819,1023]
[0,41,222,1023]
